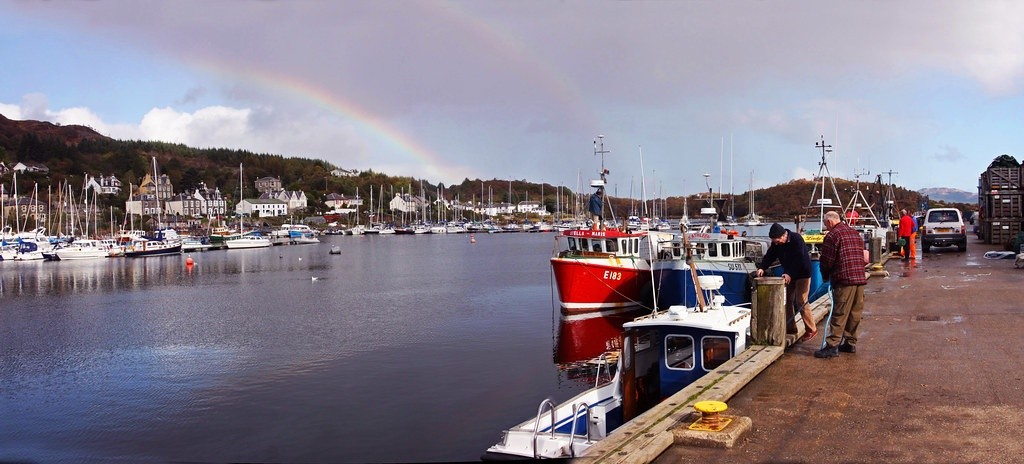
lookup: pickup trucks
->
[921,207,966,254]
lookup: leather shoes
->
[802,329,818,342]
[786,328,797,334]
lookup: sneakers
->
[838,343,856,353]
[815,345,839,357]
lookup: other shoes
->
[901,258,909,261]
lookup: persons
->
[814,211,866,358]
[590,191,602,230]
[756,223,817,342]
[908,209,918,259]
[898,209,914,261]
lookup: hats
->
[769,222,786,238]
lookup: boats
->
[327,214,670,236]
[42,238,124,260]
[124,229,185,256]
[488,185,804,457]
[225,234,273,250]
[0,230,50,261]
[183,220,319,250]
[549,228,676,312]
